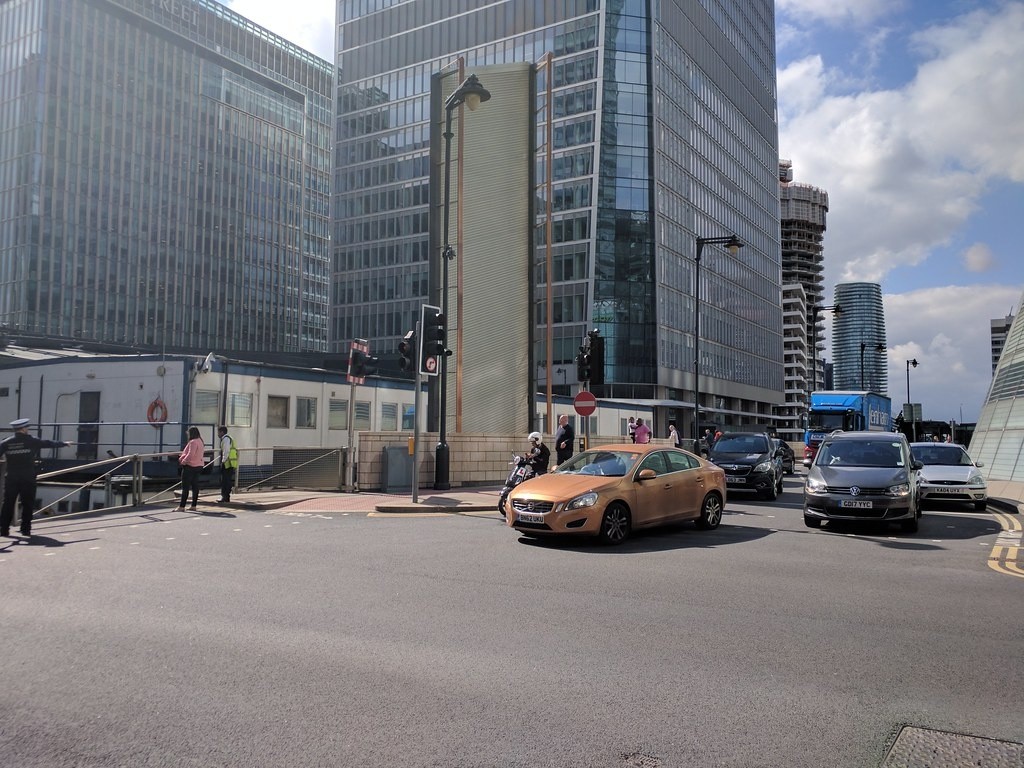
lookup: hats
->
[9,418,30,430]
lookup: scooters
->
[498,449,559,517]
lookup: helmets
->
[528,432,543,445]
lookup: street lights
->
[860,342,887,390]
[811,304,845,390]
[432,74,492,490]
[907,358,919,403]
[694,235,745,458]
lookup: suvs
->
[803,430,924,533]
[704,431,784,501]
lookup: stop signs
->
[574,391,597,416]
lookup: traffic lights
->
[577,352,593,382]
[419,303,446,375]
[350,351,380,378]
[397,339,415,373]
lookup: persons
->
[216,426,236,503]
[526,431,550,475]
[555,415,576,471]
[629,416,652,444]
[933,436,940,442]
[669,425,684,448]
[830,446,855,466]
[581,455,610,475]
[171,427,204,512]
[703,429,714,448]
[0,418,72,537]
[944,436,954,444]
[714,429,722,441]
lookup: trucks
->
[803,389,891,470]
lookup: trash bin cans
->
[682,439,694,453]
[383,446,412,494]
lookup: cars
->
[506,444,728,545]
[910,442,989,511]
[772,438,795,475]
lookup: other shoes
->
[0,531,9,537]
[172,507,185,512]
[187,506,196,511]
[21,531,30,536]
[218,498,230,502]
